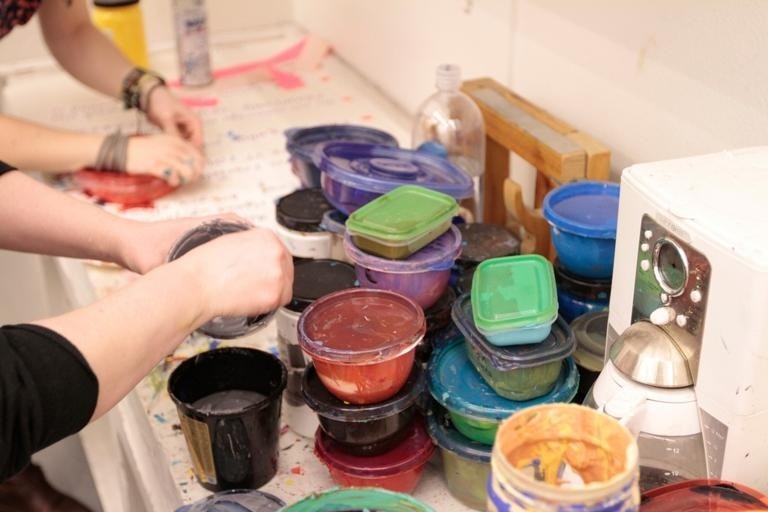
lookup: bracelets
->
[95,130,128,173]
[122,68,162,111]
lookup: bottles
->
[409,63,486,223]
[482,401,649,511]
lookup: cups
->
[165,346,289,490]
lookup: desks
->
[0,1,480,512]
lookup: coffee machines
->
[585,145,764,503]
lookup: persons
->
[0,0,207,189]
[0,161,295,512]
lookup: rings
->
[160,165,172,180]
